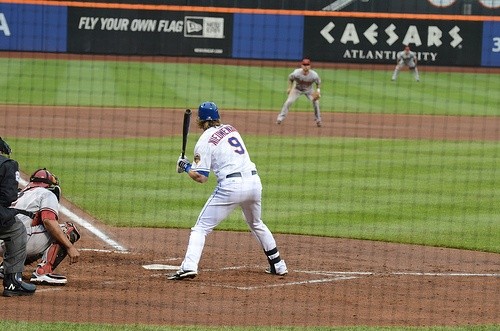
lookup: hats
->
[405,47,410,51]
[302,59,310,65]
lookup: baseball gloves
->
[309,92,320,102]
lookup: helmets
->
[26,169,53,188]
[198,101,219,121]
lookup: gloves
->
[178,156,193,173]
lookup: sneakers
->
[264,268,288,275]
[0,266,4,278]
[2,280,37,297]
[167,270,197,280]
[30,271,67,286]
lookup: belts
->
[226,170,257,178]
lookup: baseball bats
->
[181,108,192,159]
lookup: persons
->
[0,137,36,296]
[392,46,420,81]
[277,59,321,126]
[0,169,80,284]
[168,102,288,278]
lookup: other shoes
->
[316,120,322,126]
[277,120,281,125]
